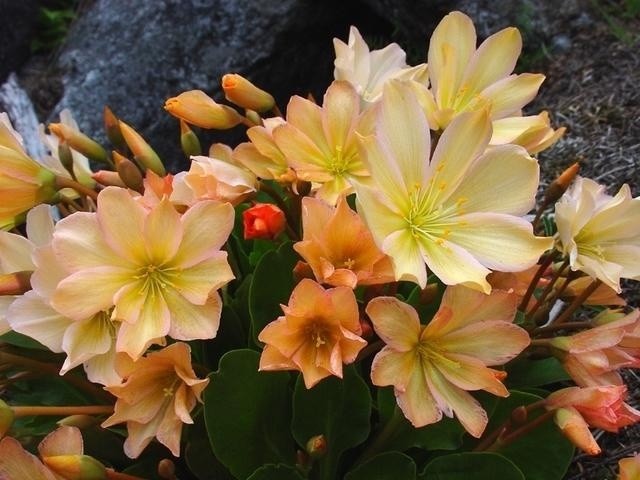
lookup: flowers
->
[0,11,640,480]
